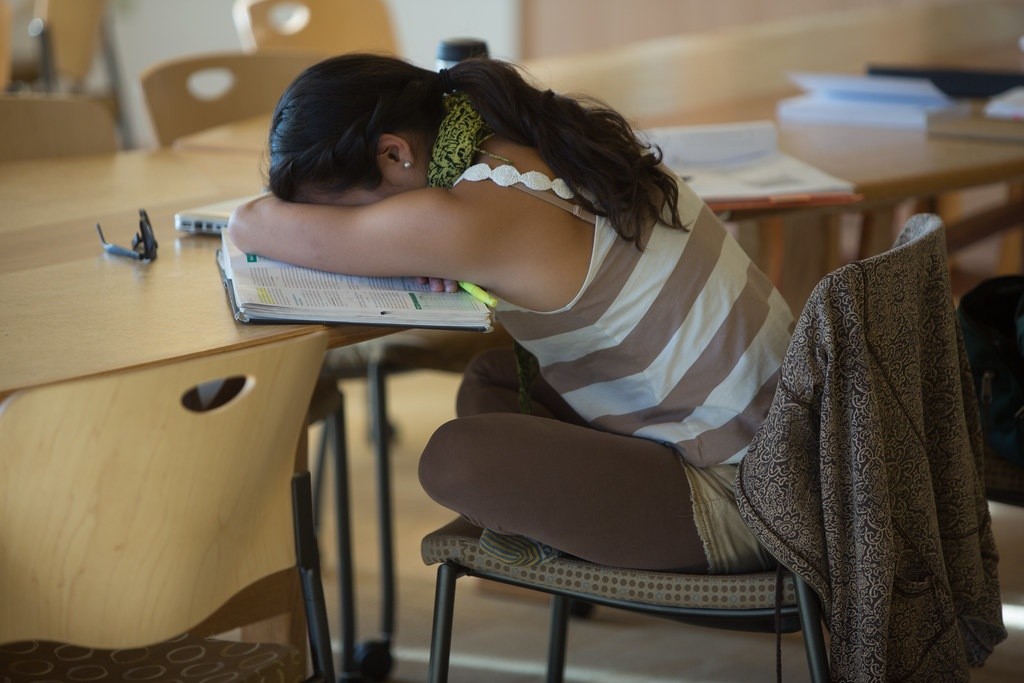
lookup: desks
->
[502,0,1024,324]
[173,0,1024,306]
[0,114,496,403]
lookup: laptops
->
[177,190,270,236]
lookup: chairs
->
[0,0,406,167]
[418,212,1010,683]
[0,327,345,683]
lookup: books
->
[639,74,1021,205]
[216,230,498,333]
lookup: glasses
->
[96,208,158,261]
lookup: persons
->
[230,51,799,573]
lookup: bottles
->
[437,37,489,73]
